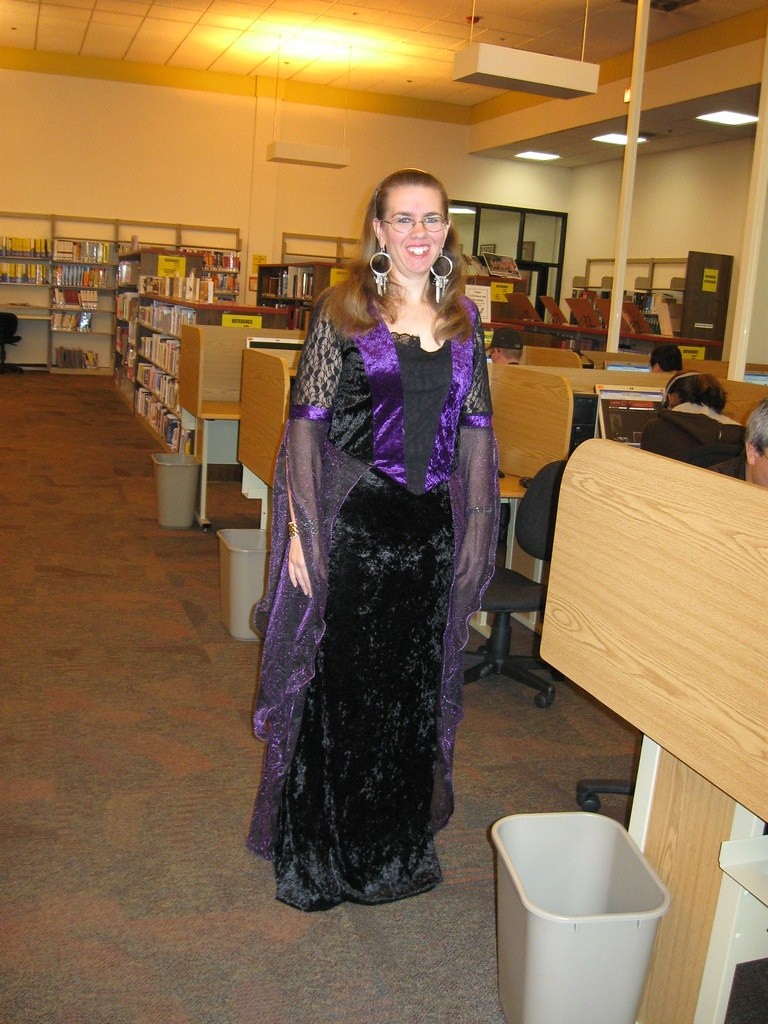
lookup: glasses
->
[381,216,448,234]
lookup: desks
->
[177,325,768,1024]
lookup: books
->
[261,266,313,330]
[54,345,99,370]
[0,236,109,288]
[633,293,677,335]
[572,288,627,310]
[115,242,239,455]
[458,254,489,277]
[53,289,97,333]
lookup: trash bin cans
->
[151,452,202,530]
[490,812,671,1024]
[217,528,267,641]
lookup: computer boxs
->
[569,388,600,459]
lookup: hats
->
[485,327,524,350]
[662,370,701,409]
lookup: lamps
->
[448,204,476,214]
[265,34,350,169]
[450,0,601,101]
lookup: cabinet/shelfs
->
[0,236,734,453]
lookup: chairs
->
[686,444,747,482]
[460,461,567,709]
[0,312,24,374]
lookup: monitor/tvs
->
[743,371,768,387]
[603,361,651,372]
[594,384,665,450]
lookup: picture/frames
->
[480,244,495,256]
[513,241,535,296]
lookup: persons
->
[706,394,768,488]
[649,344,683,374]
[244,167,500,913]
[485,327,524,365]
[640,370,747,469]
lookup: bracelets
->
[288,521,321,537]
[464,506,495,517]
